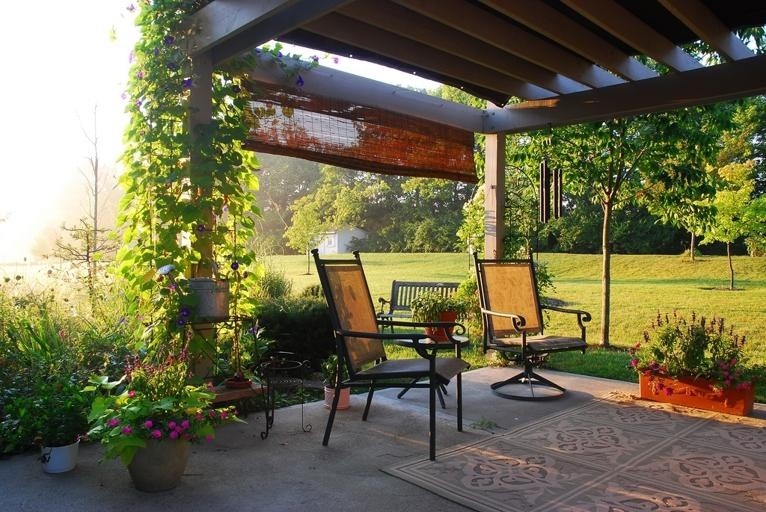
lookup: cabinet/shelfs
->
[162,313,270,439]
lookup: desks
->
[391,332,469,409]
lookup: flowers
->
[629,307,760,402]
[72,331,248,475]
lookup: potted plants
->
[409,290,466,343]
[320,355,351,410]
[1,359,90,472]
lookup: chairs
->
[473,248,591,402]
[311,247,471,461]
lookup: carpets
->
[377,390,766,512]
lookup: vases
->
[127,437,190,494]
[637,371,753,415]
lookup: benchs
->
[376,279,461,334]
